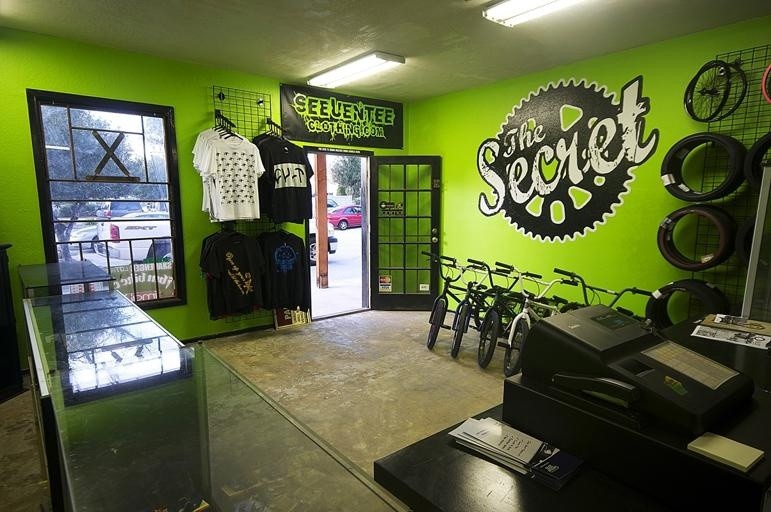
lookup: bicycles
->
[421,251,654,377]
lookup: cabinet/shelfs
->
[21,286,185,510]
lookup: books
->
[701,313,771,336]
[690,324,771,351]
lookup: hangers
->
[212,113,243,141]
[270,217,290,240]
[266,120,288,141]
[218,221,242,242]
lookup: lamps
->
[307,51,405,89]
[482,0,578,28]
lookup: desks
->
[374,308,771,512]
[17,258,117,301]
[44,337,405,511]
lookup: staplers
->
[549,370,649,431]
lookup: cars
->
[309,196,363,266]
[53,198,175,263]
